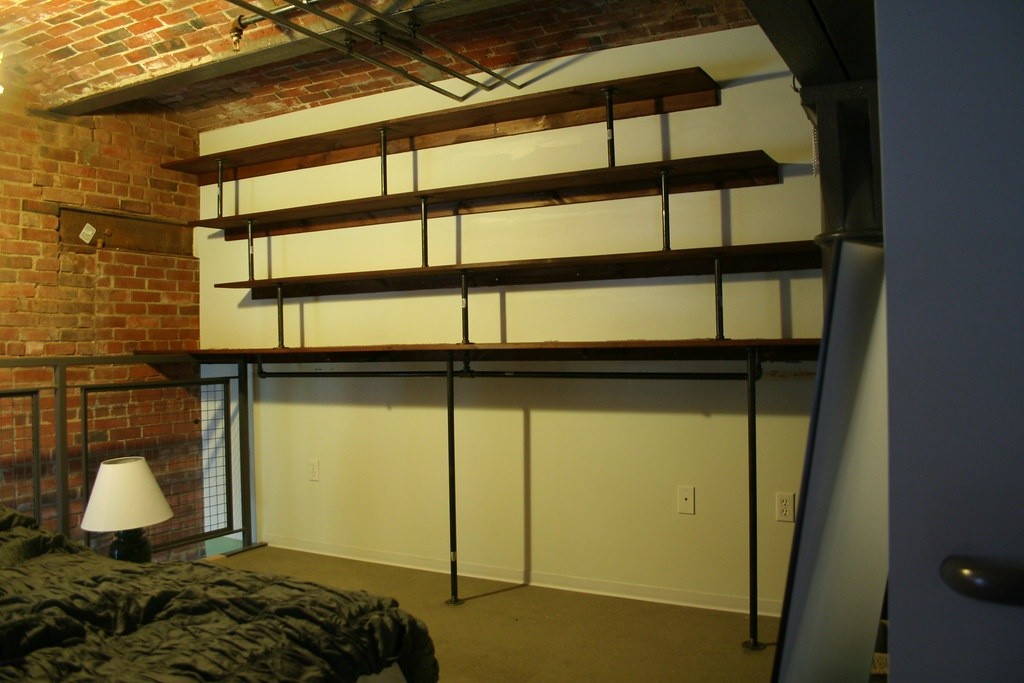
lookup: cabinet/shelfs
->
[130,67,824,365]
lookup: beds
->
[0,504,440,682]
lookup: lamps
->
[79,456,175,563]
